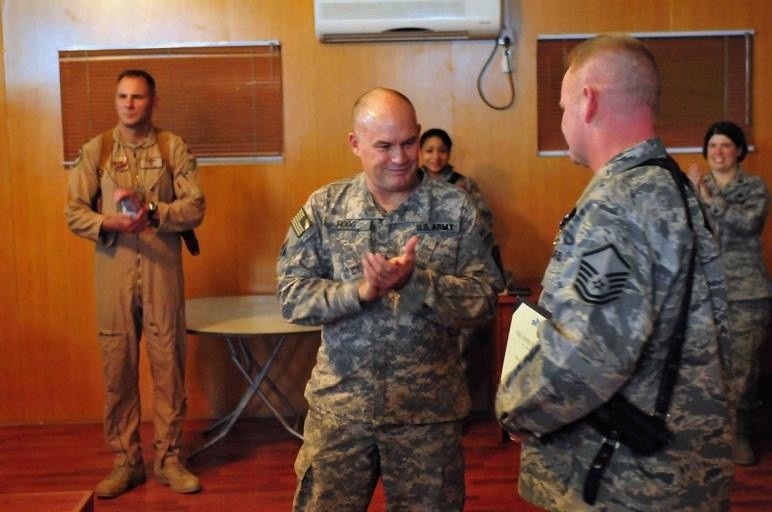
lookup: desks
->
[170,294,330,460]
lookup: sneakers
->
[734,432,756,464]
[154,457,202,493]
[95,460,146,497]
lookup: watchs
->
[146,201,157,219]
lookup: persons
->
[416,127,496,256]
[686,120,772,466]
[275,89,503,512]
[492,27,739,512]
[65,69,207,498]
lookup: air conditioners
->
[310,1,507,45]
